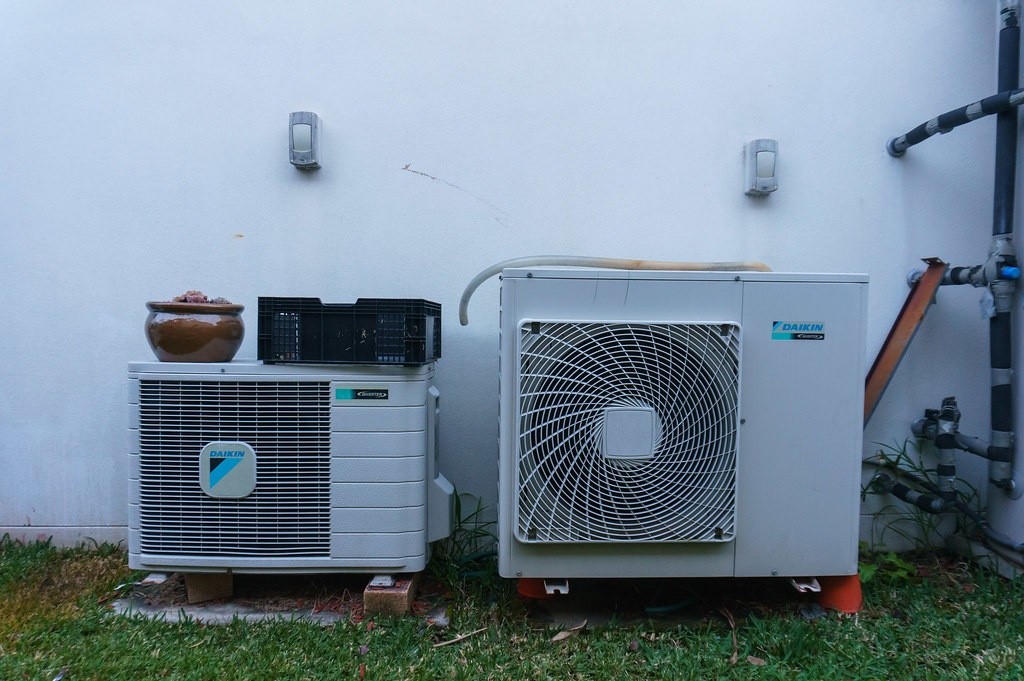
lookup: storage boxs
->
[257,296,441,367]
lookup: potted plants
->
[144,291,246,363]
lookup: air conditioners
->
[126,360,455,586]
[497,265,870,580]
